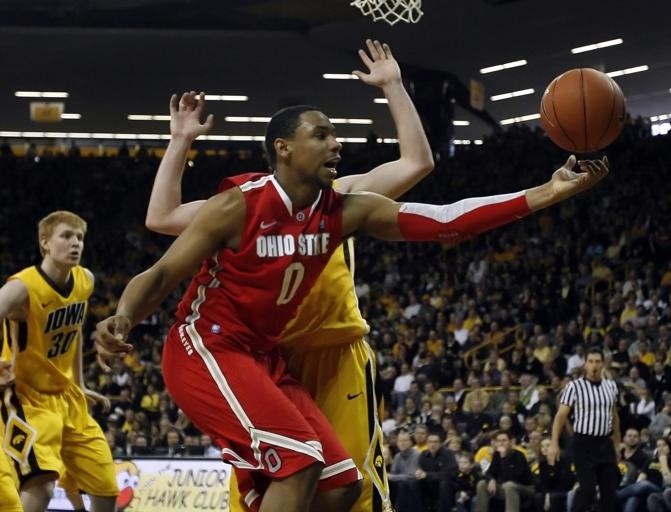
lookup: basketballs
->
[541,68,625,154]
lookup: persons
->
[138,38,440,510]
